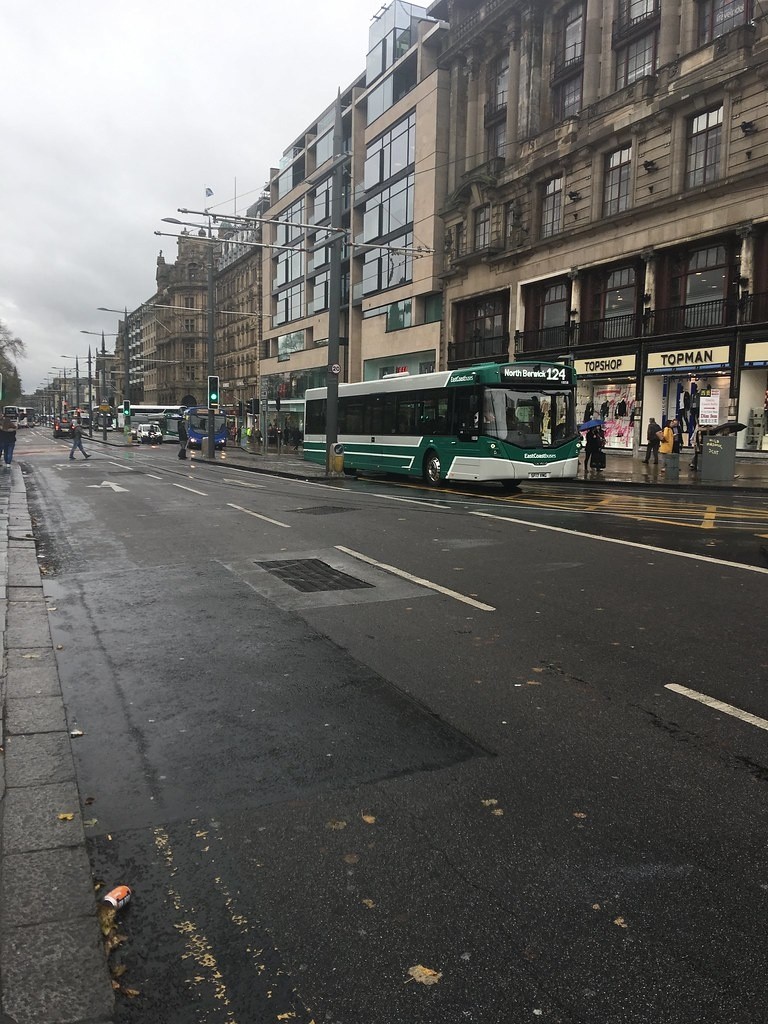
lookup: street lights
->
[96,306,154,400]
[160,217,257,457]
[36,344,96,438]
[79,329,118,440]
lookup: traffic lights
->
[123,401,131,416]
[244,399,255,414]
[207,376,219,411]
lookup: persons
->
[539,399,549,432]
[583,394,639,427]
[177,421,189,461]
[69,420,91,460]
[147,426,156,446]
[474,401,495,428]
[584,426,606,473]
[721,427,730,436]
[641,418,683,472]
[764,391,768,434]
[227,422,303,451]
[690,418,716,467]
[678,386,700,434]
[0,414,17,468]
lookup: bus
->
[183,407,227,450]
[117,405,187,429]
[303,361,583,488]
[64,410,89,428]
[133,412,181,444]
[92,406,114,431]
[2,405,35,429]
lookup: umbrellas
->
[579,419,607,432]
[712,421,747,435]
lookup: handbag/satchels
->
[590,448,606,468]
[655,429,669,440]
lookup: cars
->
[53,422,74,439]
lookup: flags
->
[206,188,214,197]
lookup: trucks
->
[130,416,148,441]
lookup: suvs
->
[137,424,163,445]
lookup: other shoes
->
[689,463,697,467]
[642,460,649,464]
[585,470,589,473]
[596,470,603,472]
[653,462,658,464]
